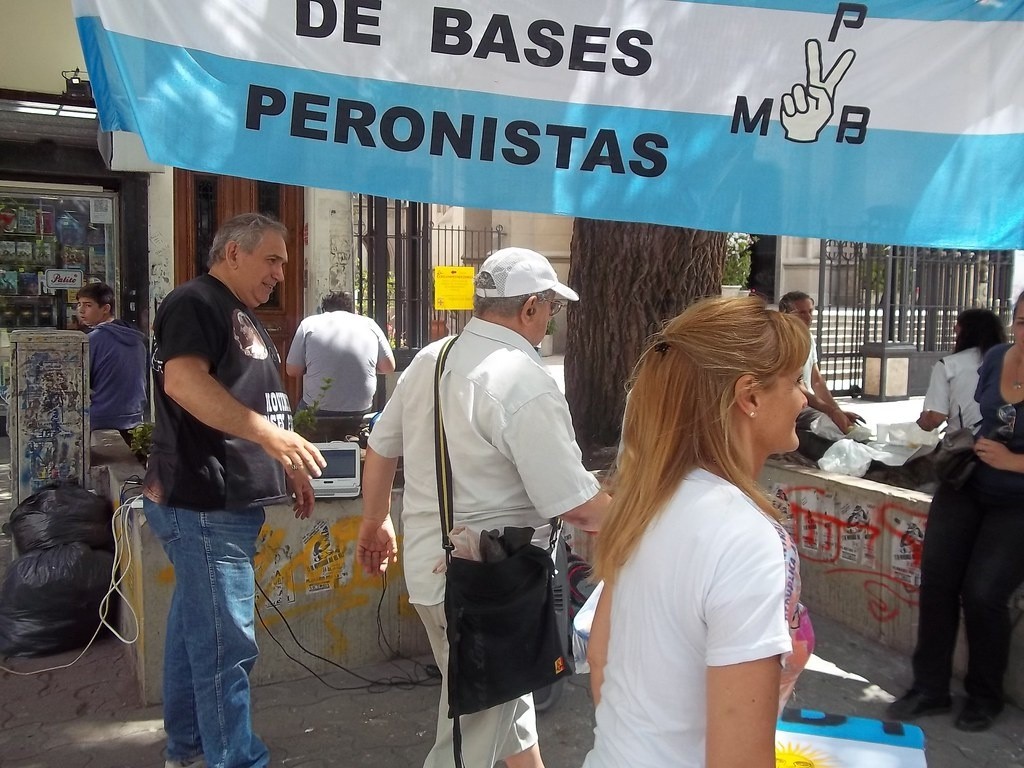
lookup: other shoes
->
[955,695,1004,732]
[165,754,205,768]
[885,683,950,719]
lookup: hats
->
[474,247,579,301]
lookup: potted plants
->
[894,258,917,309]
[861,246,886,305]
[722,252,752,300]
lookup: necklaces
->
[1013,360,1024,389]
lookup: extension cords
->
[131,500,143,508]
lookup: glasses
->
[544,298,562,316]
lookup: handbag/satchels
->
[443,544,573,720]
[932,425,980,494]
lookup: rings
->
[292,464,298,470]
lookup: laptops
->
[291,442,361,499]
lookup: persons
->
[885,287,1024,732]
[581,296,817,767]
[915,308,1007,440]
[778,290,868,462]
[286,289,396,442]
[356,247,615,768]
[72,282,149,447]
[140,212,327,768]
